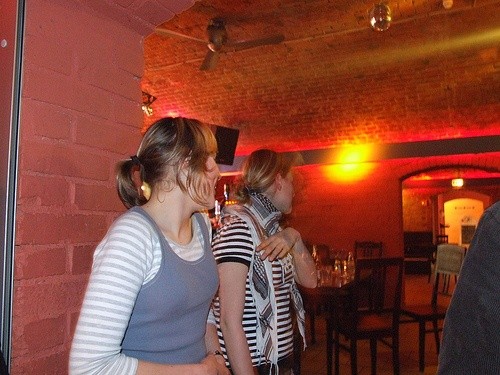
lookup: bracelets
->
[212,350,223,357]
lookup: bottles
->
[348,252,355,281]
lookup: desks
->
[299,277,375,375]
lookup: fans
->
[155,19,285,71]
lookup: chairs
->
[325,241,467,375]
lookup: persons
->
[66,116,232,375]
[211,149,318,375]
[435,201,500,375]
